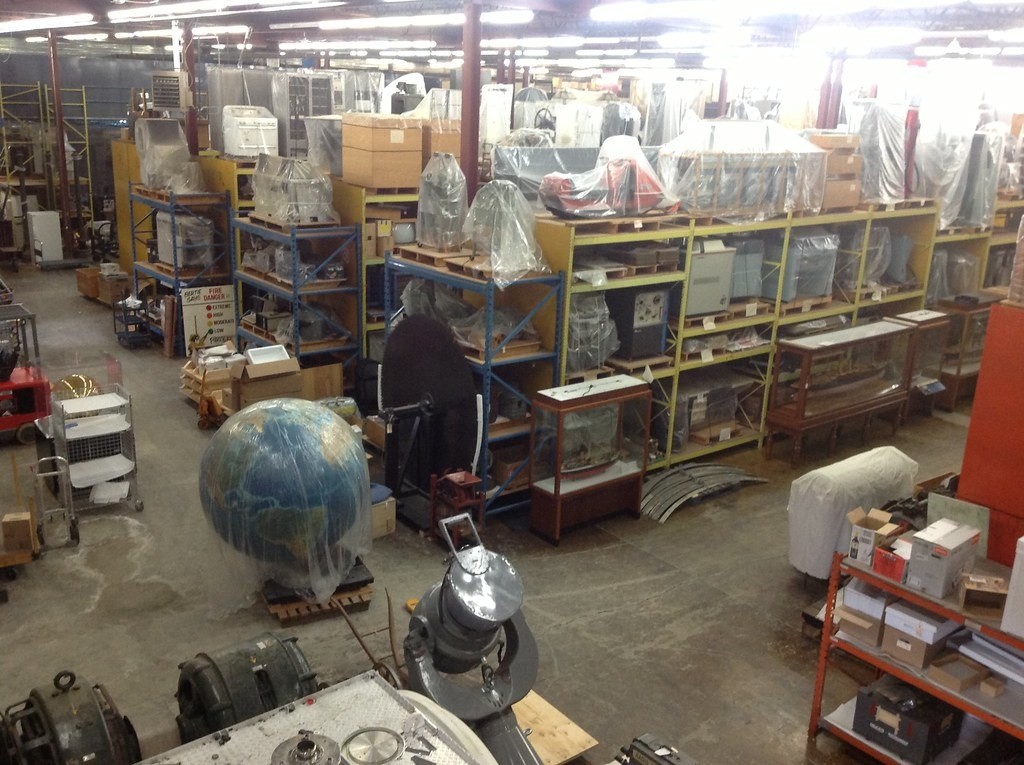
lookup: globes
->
[196,395,376,605]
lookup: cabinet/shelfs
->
[109,137,157,298]
[331,176,423,360]
[528,372,651,543]
[764,318,917,470]
[878,308,952,433]
[462,195,940,479]
[127,181,235,357]
[51,382,146,525]
[924,284,1012,415]
[112,299,149,351]
[950,197,1024,301]
[655,144,825,227]
[187,153,258,351]
[383,238,568,527]
[801,131,866,216]
[804,495,1024,765]
[230,208,363,397]
[921,223,990,310]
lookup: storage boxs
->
[906,516,983,601]
[998,536,1024,640]
[843,506,901,569]
[1,511,36,554]
[958,571,1008,622]
[833,599,1024,698]
[26,210,63,266]
[240,358,302,381]
[871,528,914,586]
[32,409,126,499]
[842,577,900,621]
[851,672,965,765]
[341,114,424,194]
[76,267,104,299]
[426,118,462,173]
[95,271,129,308]
